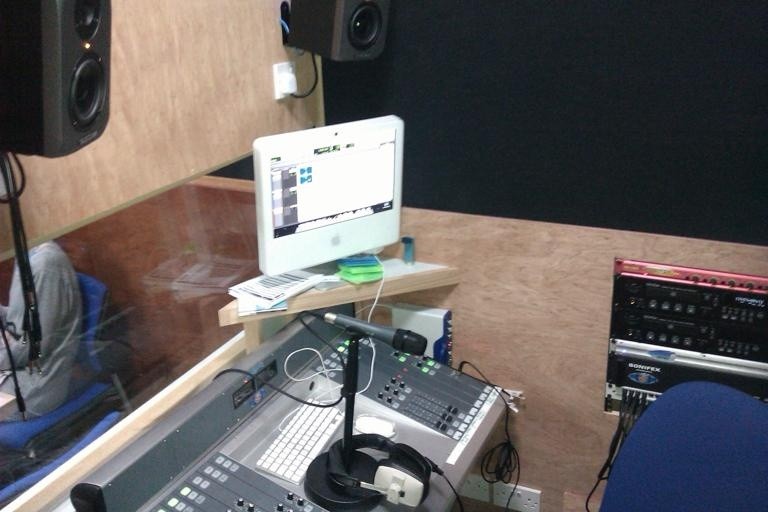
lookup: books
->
[335,256,383,285]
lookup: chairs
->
[0,271,133,462]
[599,380,768,512]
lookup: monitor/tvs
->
[252,114,405,277]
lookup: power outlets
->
[459,474,541,512]
[272,60,298,103]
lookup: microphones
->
[304,309,427,357]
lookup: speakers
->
[287,1,391,62]
[0,0,111,157]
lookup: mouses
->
[354,415,396,439]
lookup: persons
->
[0,240,85,422]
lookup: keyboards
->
[255,399,345,486]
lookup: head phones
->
[326,434,430,508]
